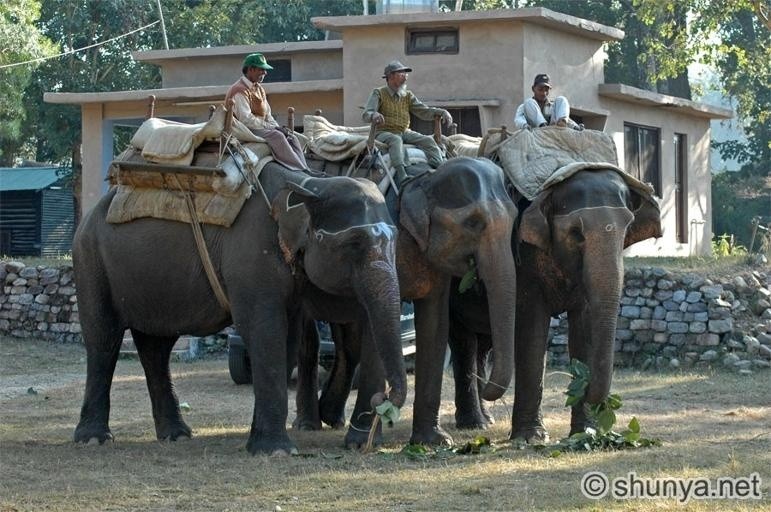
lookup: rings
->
[376,120,379,123]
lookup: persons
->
[513,73,585,131]
[361,60,453,183]
[225,54,327,178]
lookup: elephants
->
[71,101,663,460]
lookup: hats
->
[383,60,412,77]
[242,53,274,71]
[533,73,553,90]
